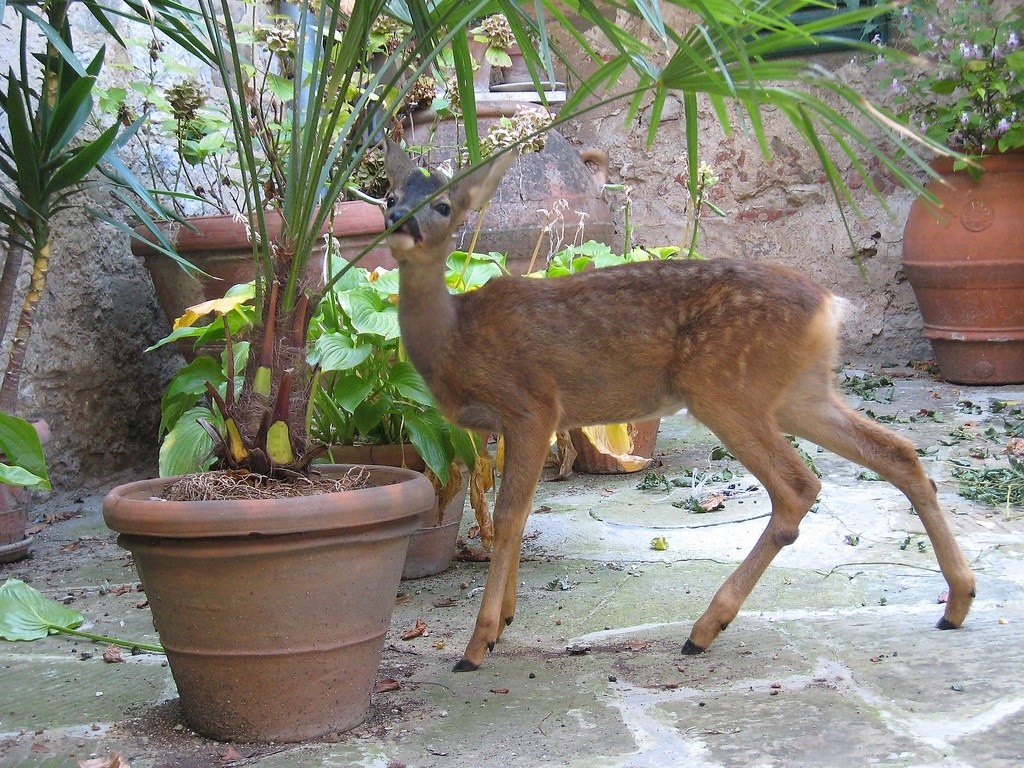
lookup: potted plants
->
[1,0,986,744]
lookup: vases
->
[902,147,1024,387]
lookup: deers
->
[380,125,977,674]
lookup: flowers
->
[850,1,1023,182]
